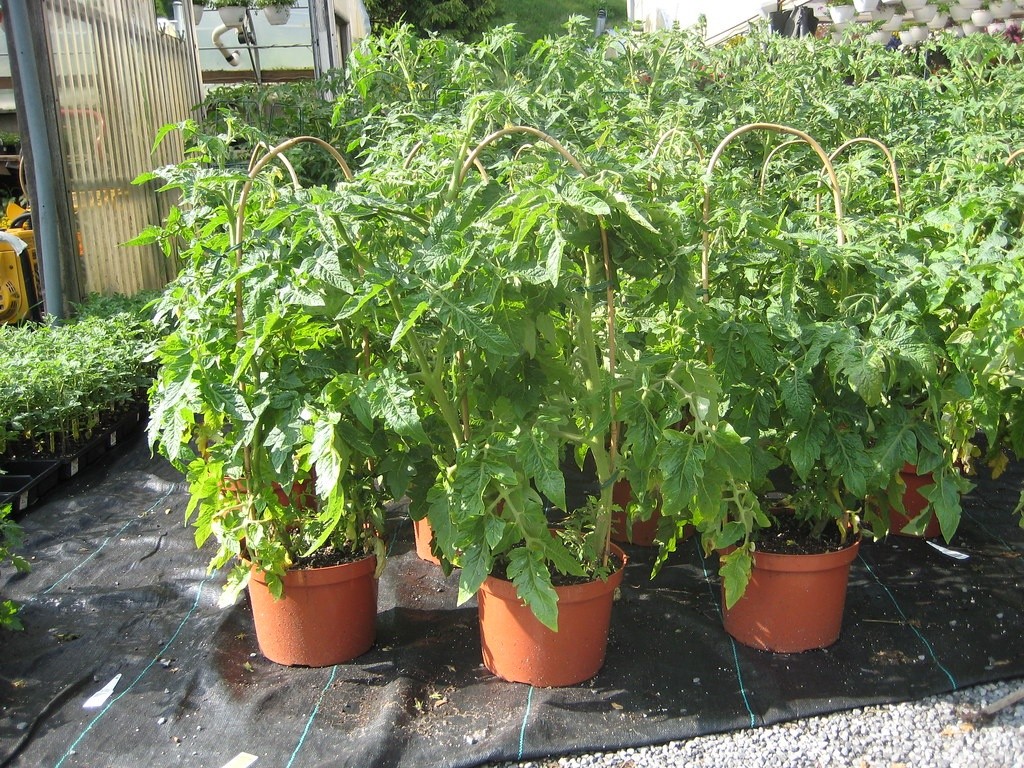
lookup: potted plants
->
[117,0,1024,690]
